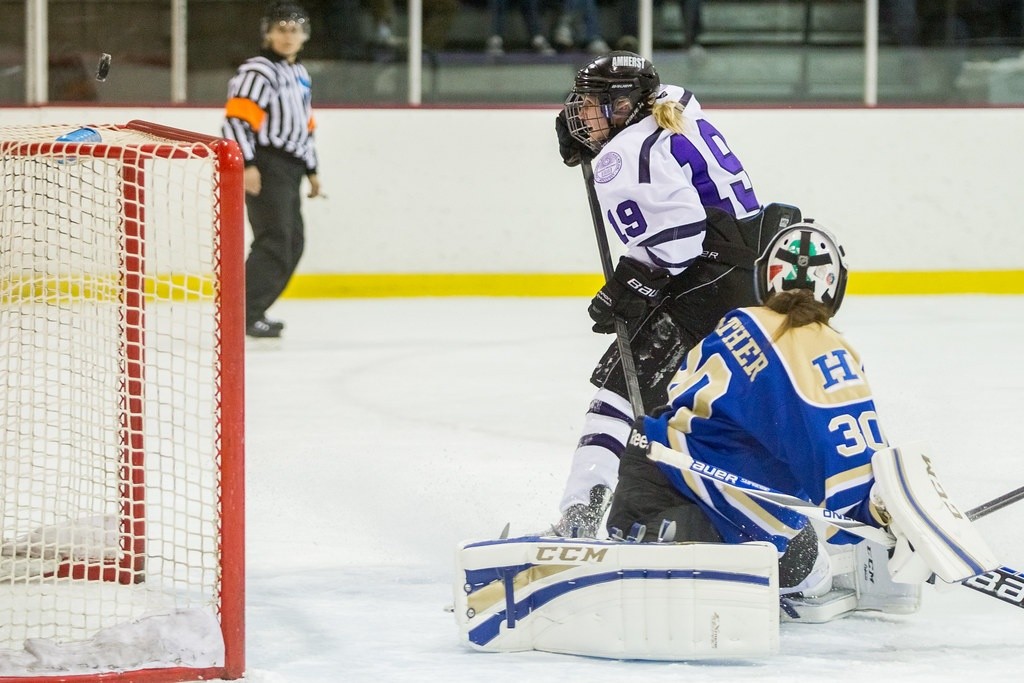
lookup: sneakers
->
[246,320,284,338]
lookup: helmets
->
[754,219,848,315]
[571,50,661,118]
[261,2,311,32]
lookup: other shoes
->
[540,484,615,538]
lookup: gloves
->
[589,256,671,333]
[555,94,598,167]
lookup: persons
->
[606,218,890,596]
[221,0,318,338]
[618,0,706,56]
[524,50,762,539]
[552,0,611,57]
[482,0,557,59]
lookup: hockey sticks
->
[580,159,646,420]
[645,441,1024,608]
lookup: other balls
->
[95,53,112,82]
